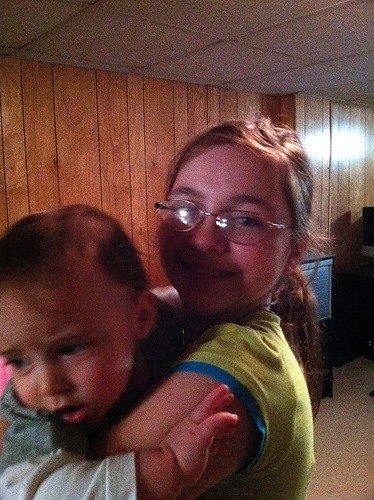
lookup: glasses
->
[154,198,294,247]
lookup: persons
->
[0,116,332,500]
[0,202,239,500]
